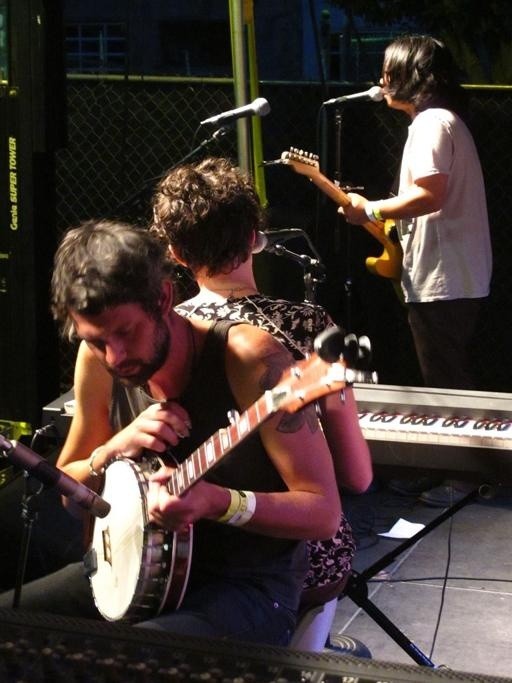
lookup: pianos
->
[40,380,512,475]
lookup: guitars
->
[257,145,402,280]
[83,323,378,621]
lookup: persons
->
[147,155,373,649]
[338,37,493,508]
[49,220,343,647]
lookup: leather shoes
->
[390,473,463,506]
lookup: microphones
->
[3,441,111,519]
[479,484,504,500]
[321,85,384,111]
[251,227,304,256]
[200,97,271,127]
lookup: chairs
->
[63,470,350,648]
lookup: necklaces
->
[206,288,259,294]
[186,317,195,368]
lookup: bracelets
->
[216,488,256,527]
[373,199,383,220]
[89,446,113,476]
[365,200,377,222]
